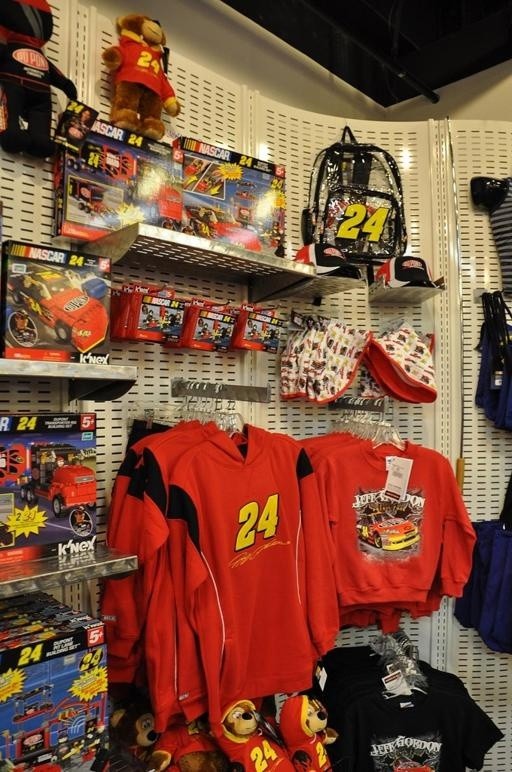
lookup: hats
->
[280,319,439,404]
[294,243,358,277]
[377,256,437,289]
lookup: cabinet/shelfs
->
[0,222,446,606]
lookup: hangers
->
[366,625,435,705]
[325,390,411,455]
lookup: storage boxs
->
[52,114,286,262]
[0,238,283,364]
[1,590,108,771]
[1,412,96,570]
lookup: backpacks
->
[301,127,407,263]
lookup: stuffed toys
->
[209,698,297,771]
[99,12,182,141]
[146,710,229,771]
[110,703,183,771]
[0,1,79,161]
[279,693,338,772]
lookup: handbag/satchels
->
[454,475,512,653]
[475,291,511,430]
